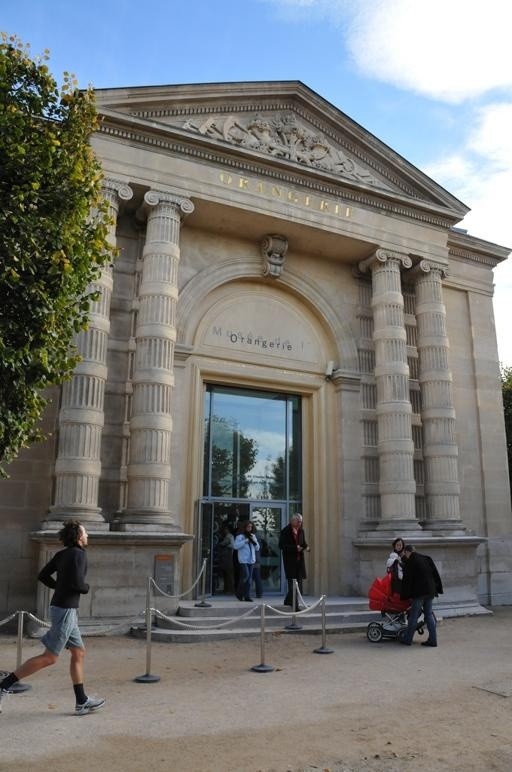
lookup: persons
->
[218,524,235,594]
[233,520,260,602]
[248,550,264,598]
[398,543,443,647]
[386,538,405,595]
[1,518,107,716]
[278,512,312,612]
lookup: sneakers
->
[75,697,105,714]
[236,593,253,602]
[0,688,13,712]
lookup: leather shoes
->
[400,639,411,645]
[421,640,436,646]
[284,600,301,612]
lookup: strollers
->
[366,572,428,644]
